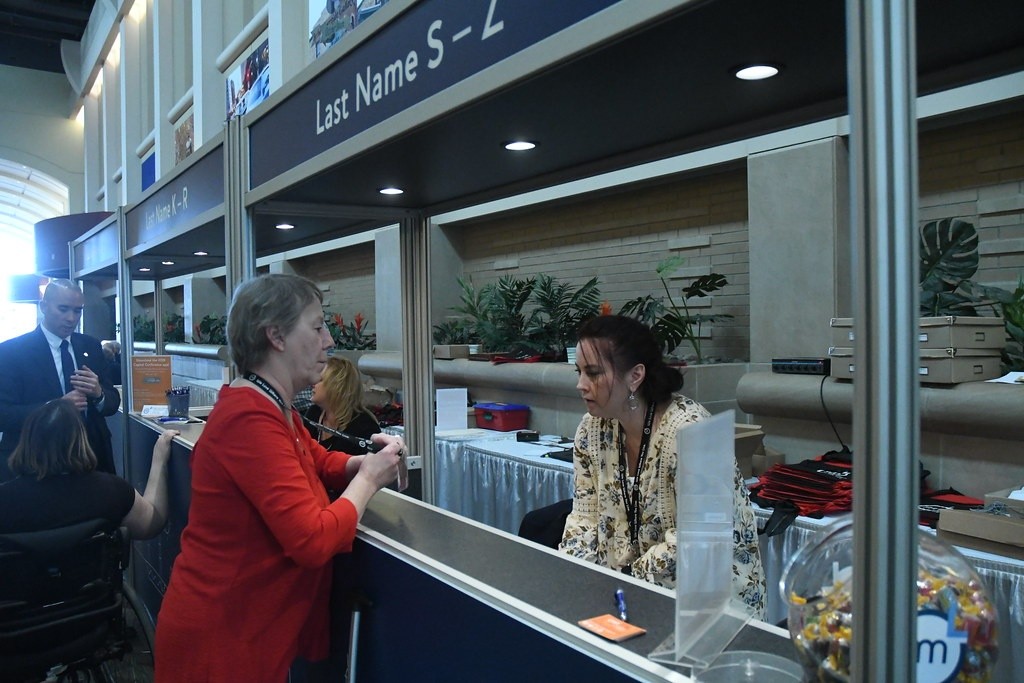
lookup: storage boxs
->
[473,403,529,431]
[937,507,1024,562]
[829,316,1006,349]
[432,344,482,359]
[828,347,1000,384]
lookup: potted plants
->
[559,304,600,364]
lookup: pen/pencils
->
[158,417,187,421]
[165,385,190,417]
[611,586,628,620]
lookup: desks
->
[187,378,476,439]
[465,433,573,536]
[744,477,1024,683]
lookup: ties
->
[60,339,76,395]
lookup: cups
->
[167,394,190,417]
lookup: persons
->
[556,315,768,622]
[100,338,122,385]
[303,357,382,460]
[1,280,122,479]
[150,274,408,683]
[0,397,182,683]
